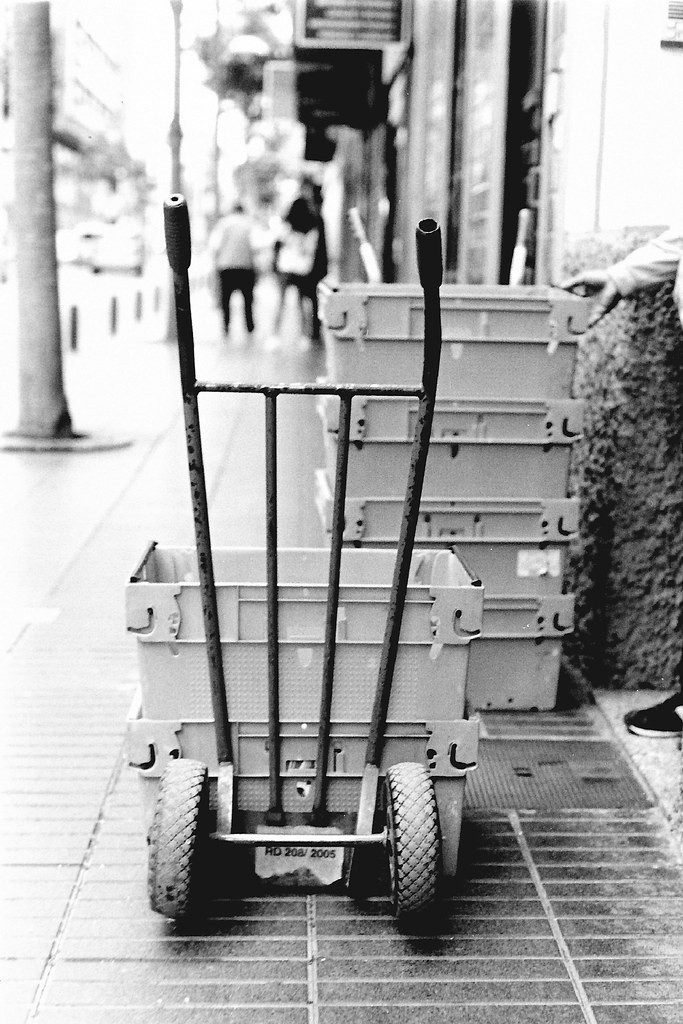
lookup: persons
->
[208,203,256,340]
[561,220,683,737]
[267,194,328,352]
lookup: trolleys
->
[126,193,485,920]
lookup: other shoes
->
[623,693,683,737]
